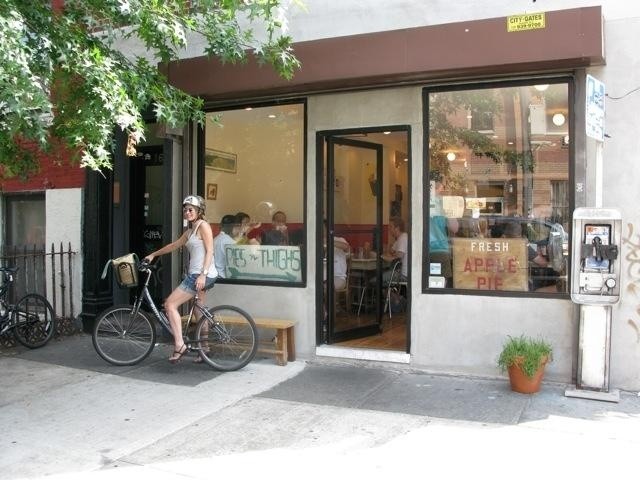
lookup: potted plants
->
[496,332,554,394]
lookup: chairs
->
[383,258,407,319]
[335,258,350,318]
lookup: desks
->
[350,259,377,318]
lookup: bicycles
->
[92,258,258,371]
[1,266,55,348]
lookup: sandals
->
[193,350,209,363]
[168,343,188,362]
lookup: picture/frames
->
[207,183,217,200]
[205,148,237,173]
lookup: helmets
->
[182,195,206,210]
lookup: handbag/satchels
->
[111,252,138,287]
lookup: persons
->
[429,170,562,292]
[371,217,408,312]
[503,222,546,266]
[145,195,218,363]
[232,212,261,244]
[322,219,350,332]
[260,211,300,245]
[213,215,240,279]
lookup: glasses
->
[183,208,194,212]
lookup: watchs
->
[200,269,208,275]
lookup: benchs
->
[182,314,298,366]
